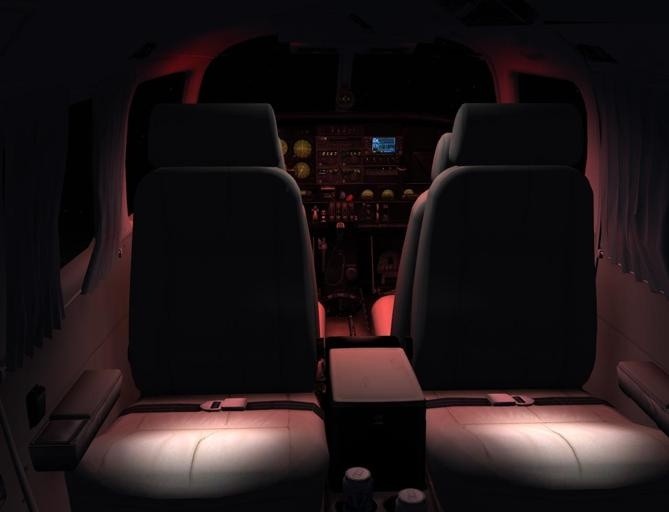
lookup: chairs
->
[370,105,669,510]
[78,102,331,508]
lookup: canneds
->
[340,466,375,512]
[394,487,427,512]
